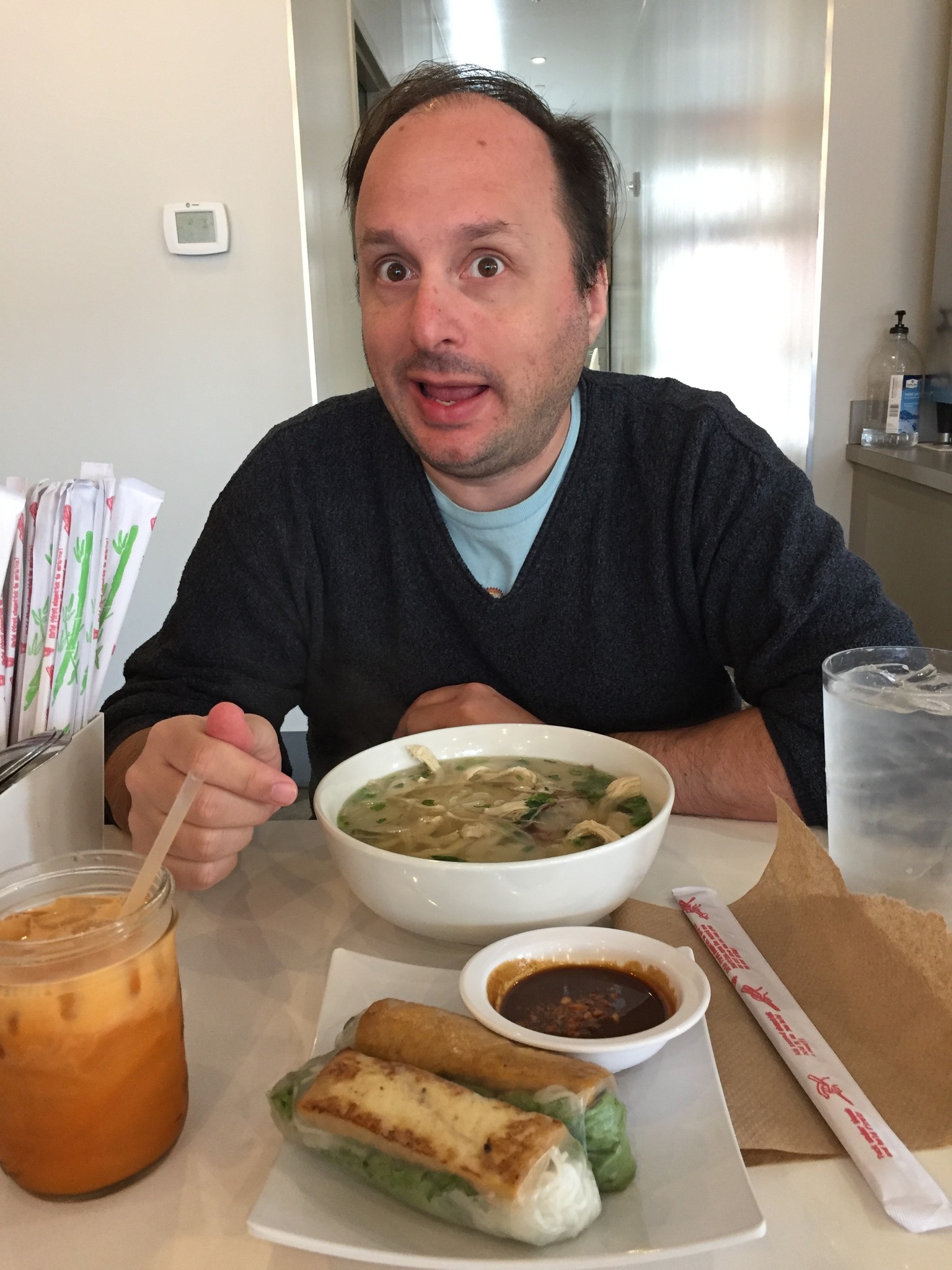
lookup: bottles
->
[859,309,924,451]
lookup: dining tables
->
[13,809,952,1270]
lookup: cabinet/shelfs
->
[846,437,952,655]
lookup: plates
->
[245,949,768,1268]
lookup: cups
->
[0,848,190,1204]
[823,644,951,925]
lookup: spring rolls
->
[337,998,638,1197]
[263,1049,603,1239]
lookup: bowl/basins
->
[313,723,677,945]
[459,925,712,1072]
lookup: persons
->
[84,55,930,900]
